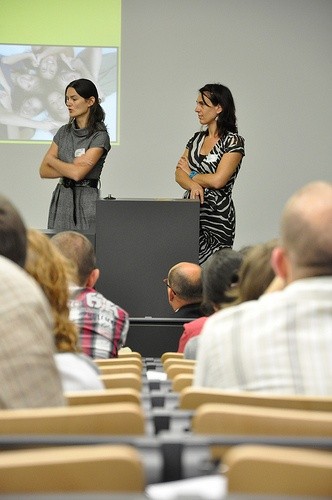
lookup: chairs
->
[1,351,147,499]
[162,351,331,497]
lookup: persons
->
[0,181,332,412]
[174,83,245,266]
[0,45,116,141]
[39,78,111,233]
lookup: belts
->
[60,177,98,226]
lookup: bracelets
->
[189,171,197,178]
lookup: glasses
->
[163,279,176,295]
